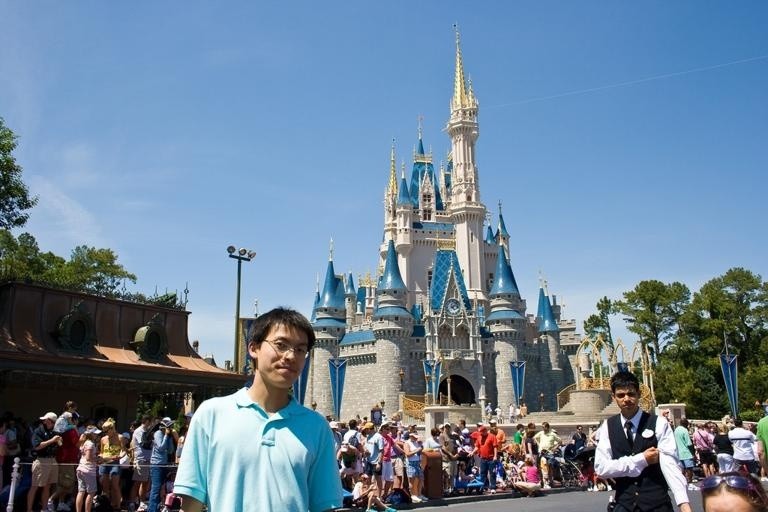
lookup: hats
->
[329,421,339,428]
[84,427,103,434]
[477,419,497,433]
[361,422,374,431]
[439,422,450,429]
[409,432,421,438]
[39,412,57,422]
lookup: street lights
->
[531,332,548,411]
[227,245,256,374]
[398,368,405,392]
[424,373,431,406]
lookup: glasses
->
[264,339,309,359]
[700,475,765,502]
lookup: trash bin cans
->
[420,452,442,499]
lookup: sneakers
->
[47,499,70,511]
[365,507,377,511]
[688,484,701,491]
[381,507,396,512]
[411,494,429,502]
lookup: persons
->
[594,370,696,510]
[172,304,347,511]
[0,400,768,512]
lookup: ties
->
[625,421,634,449]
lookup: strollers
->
[450,465,483,497]
[538,444,608,492]
[499,451,535,497]
[157,472,182,512]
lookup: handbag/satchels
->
[91,495,113,511]
